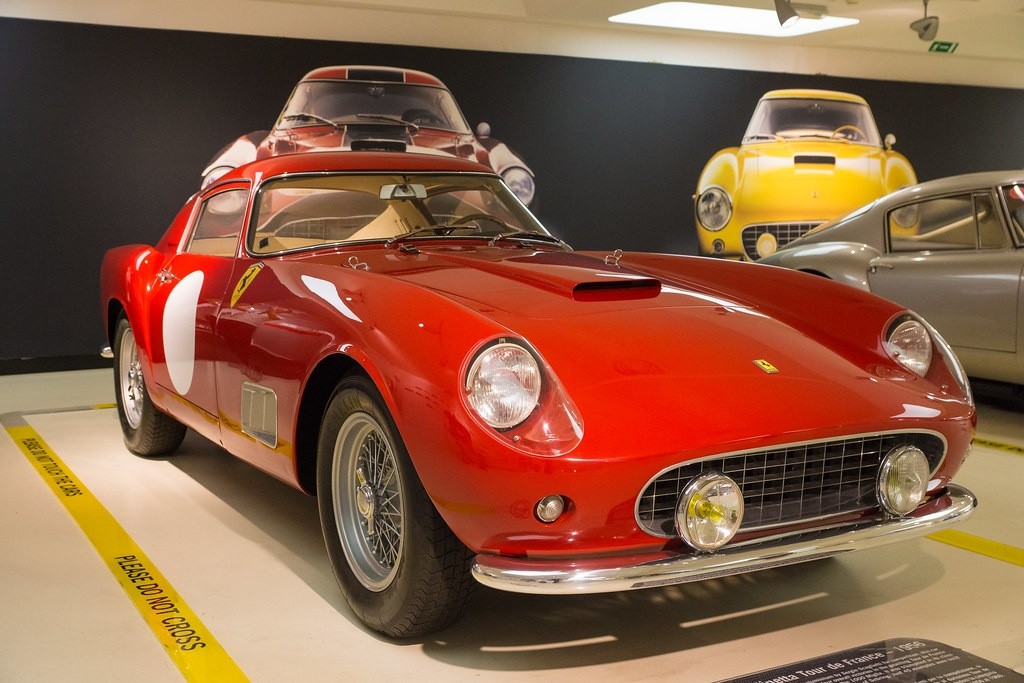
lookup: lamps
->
[774,0,799,28]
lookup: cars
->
[690,88,921,264]
[754,169,1024,389]
[97,139,976,644]
[197,67,536,232]
